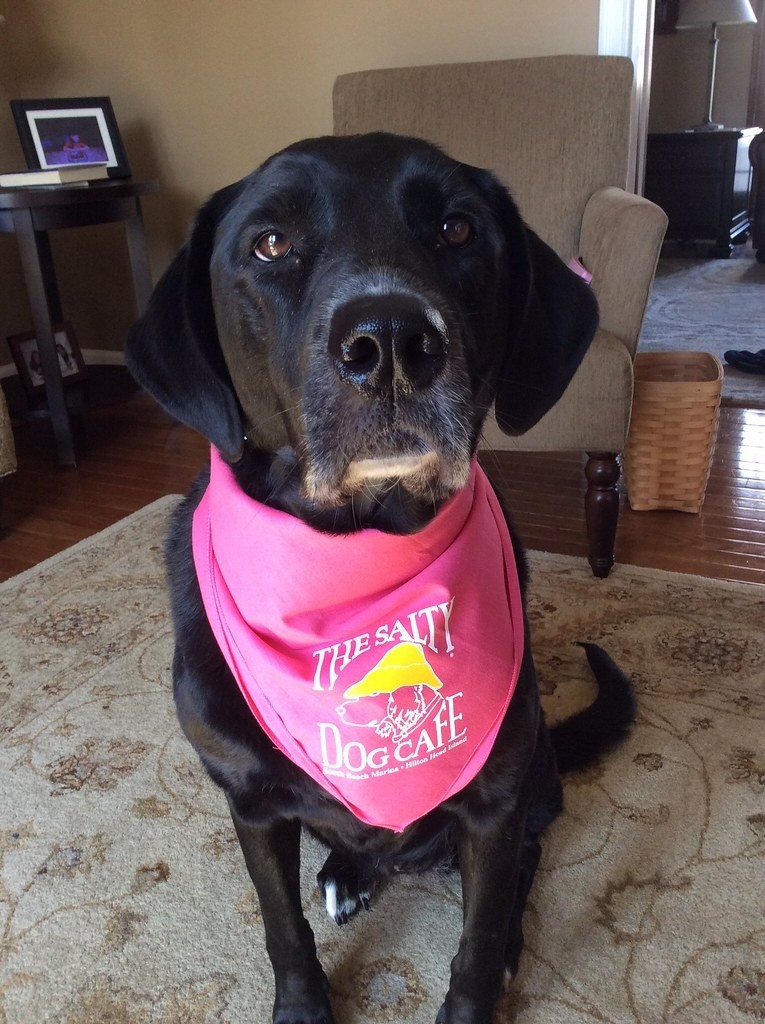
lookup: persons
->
[63,129,90,162]
[26,343,77,384]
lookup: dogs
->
[121,132,637,1024]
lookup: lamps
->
[673,0,758,130]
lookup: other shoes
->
[724,349,765,374]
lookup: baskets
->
[621,351,724,514]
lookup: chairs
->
[321,54,671,580]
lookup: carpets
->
[632,256,765,410]
[0,493,765,1024]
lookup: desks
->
[0,174,166,471]
[646,130,743,256]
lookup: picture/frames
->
[9,95,133,184]
[5,319,92,400]
